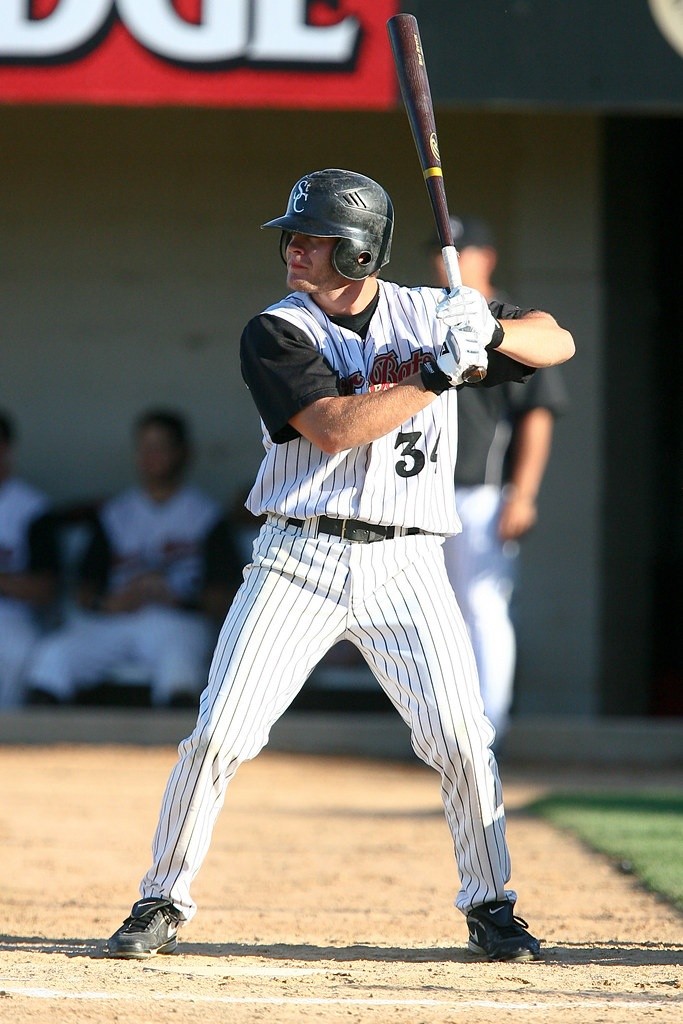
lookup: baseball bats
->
[387,12,488,384]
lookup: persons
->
[0,402,217,716]
[433,215,569,762]
[108,166,575,967]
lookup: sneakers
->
[467,901,541,964]
[106,897,179,958]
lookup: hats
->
[437,217,501,254]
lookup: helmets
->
[260,168,396,281]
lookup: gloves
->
[420,325,490,396]
[433,286,514,355]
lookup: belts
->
[290,515,418,548]
